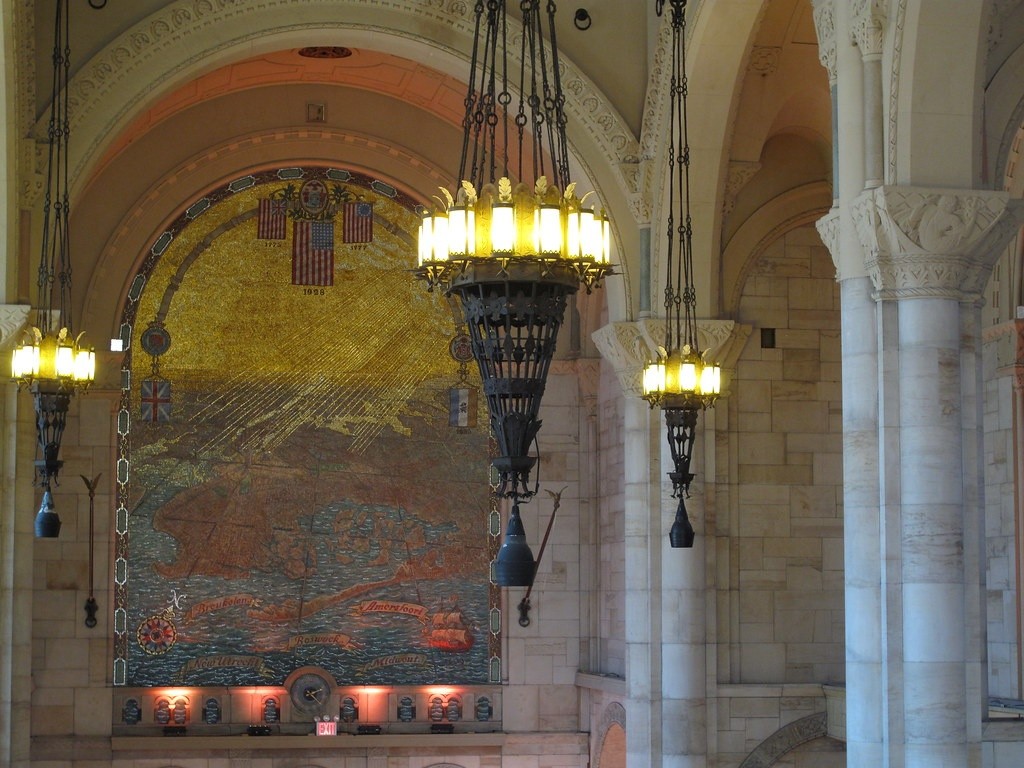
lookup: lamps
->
[11,0,97,538]
[313,712,340,736]
[165,725,187,737]
[401,0,627,588]
[641,0,721,550]
[357,724,382,735]
[246,725,272,735]
[430,722,454,735]
